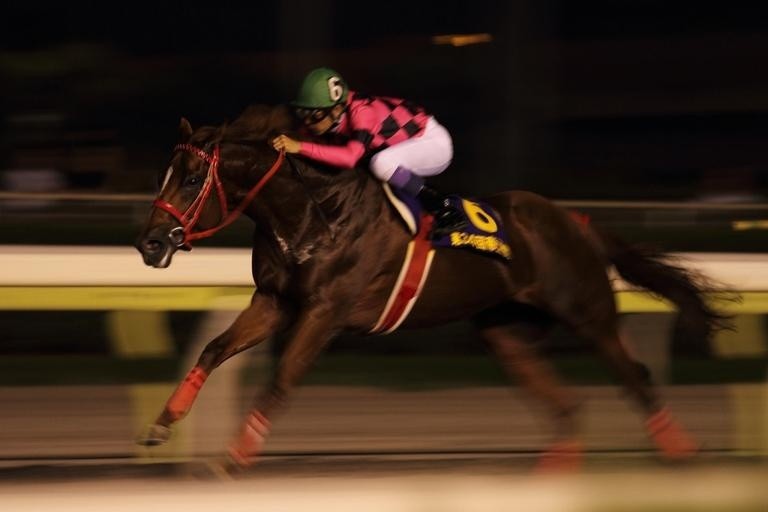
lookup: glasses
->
[296,108,330,120]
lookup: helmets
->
[290,66,348,108]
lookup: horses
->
[134,116,749,479]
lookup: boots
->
[418,183,461,228]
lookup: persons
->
[270,65,471,240]
